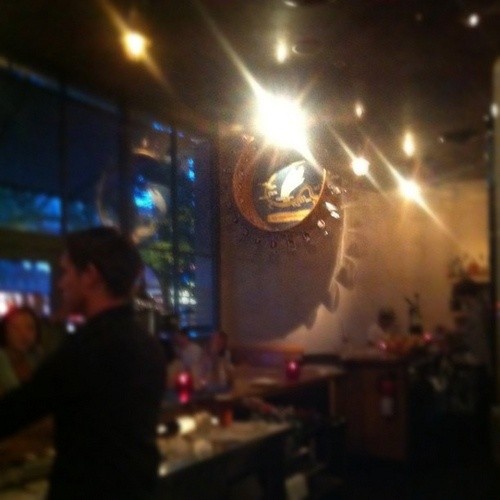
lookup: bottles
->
[210,331,232,394]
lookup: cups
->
[283,347,304,381]
[215,395,233,430]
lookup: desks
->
[0,337,454,500]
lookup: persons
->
[170,311,246,431]
[348,301,494,483]
[36,286,78,358]
[2,222,168,500]
[1,305,53,428]
